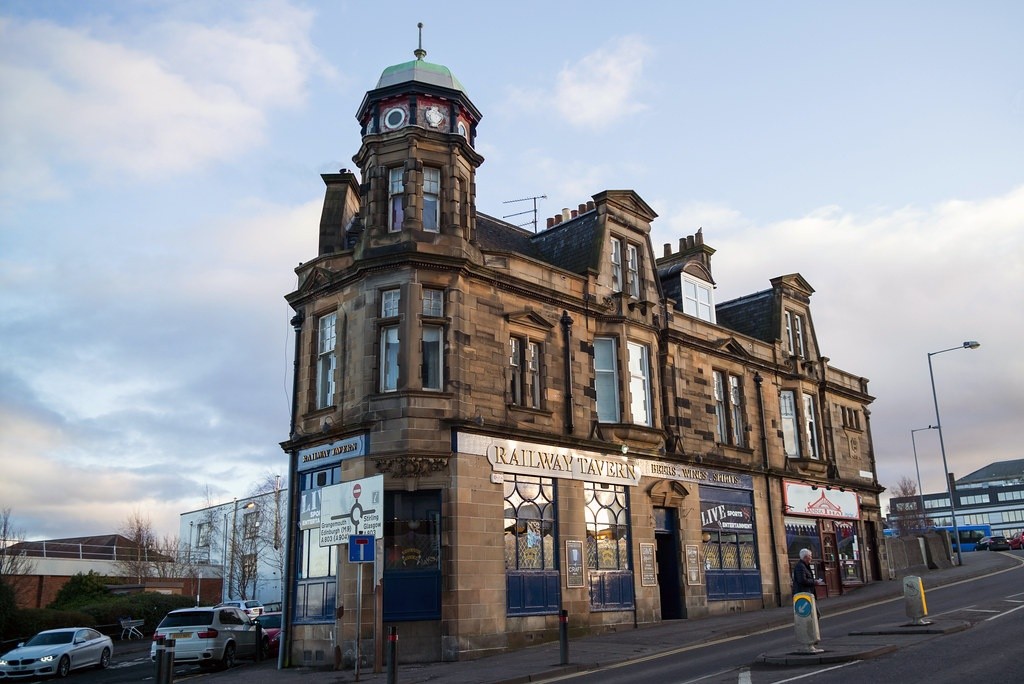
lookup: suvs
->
[224,600,266,621]
[150,603,270,673]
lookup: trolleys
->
[120,617,145,641]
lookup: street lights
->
[912,425,943,528]
[926,340,981,566]
[222,502,254,602]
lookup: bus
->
[883,524,992,553]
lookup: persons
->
[791,549,821,646]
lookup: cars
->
[253,612,282,659]
[0,628,113,681]
[1009,532,1024,550]
[972,536,1010,552]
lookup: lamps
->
[852,488,859,492]
[826,485,832,490]
[322,416,341,433]
[292,425,312,443]
[473,408,484,426]
[690,450,702,464]
[607,437,629,455]
[657,441,666,455]
[840,487,845,492]
[802,480,818,490]
